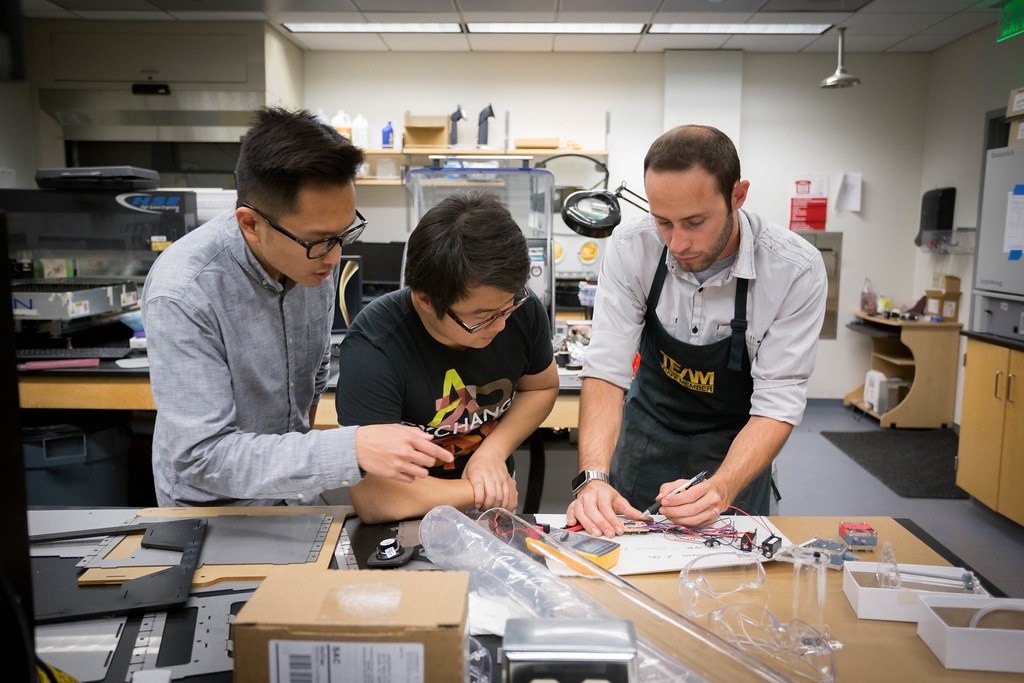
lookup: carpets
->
[822,430,970,501]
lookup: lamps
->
[561,180,650,238]
[821,25,860,90]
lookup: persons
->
[567,124,827,539]
[141,106,454,510]
[334,191,559,523]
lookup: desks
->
[18,374,582,516]
[28,507,1024,683]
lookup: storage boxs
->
[403,113,450,148]
[1005,85,1024,119]
[917,594,1024,673]
[1008,119,1024,147]
[932,274,961,293]
[840,560,991,621]
[924,290,963,321]
[515,136,560,149]
[230,564,472,683]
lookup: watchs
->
[571,470,609,499]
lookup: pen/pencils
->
[641,470,709,518]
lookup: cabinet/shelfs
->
[353,147,610,189]
[842,305,962,429]
[955,329,1024,529]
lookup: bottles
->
[860,278,876,317]
[316,108,394,149]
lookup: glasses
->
[243,201,368,259]
[679,551,772,618]
[469,637,492,683]
[710,603,836,683]
[446,286,531,334]
